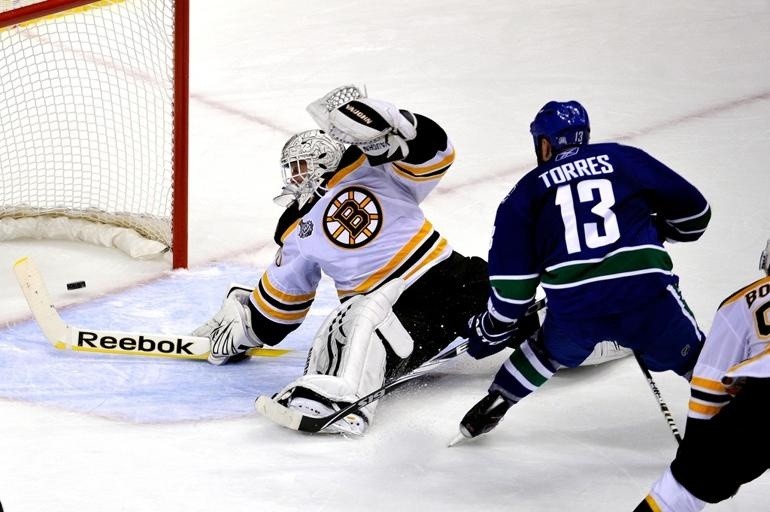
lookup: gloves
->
[305,82,417,167]
[466,310,520,360]
[189,287,263,367]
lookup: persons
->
[460,102,706,440]
[635,241,770,512]
[192,98,542,433]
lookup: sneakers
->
[254,375,370,437]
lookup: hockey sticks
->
[14,257,309,358]
[254,297,549,433]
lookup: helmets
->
[530,100,591,167]
[759,238,770,275]
[281,129,346,174]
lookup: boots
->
[459,390,509,438]
